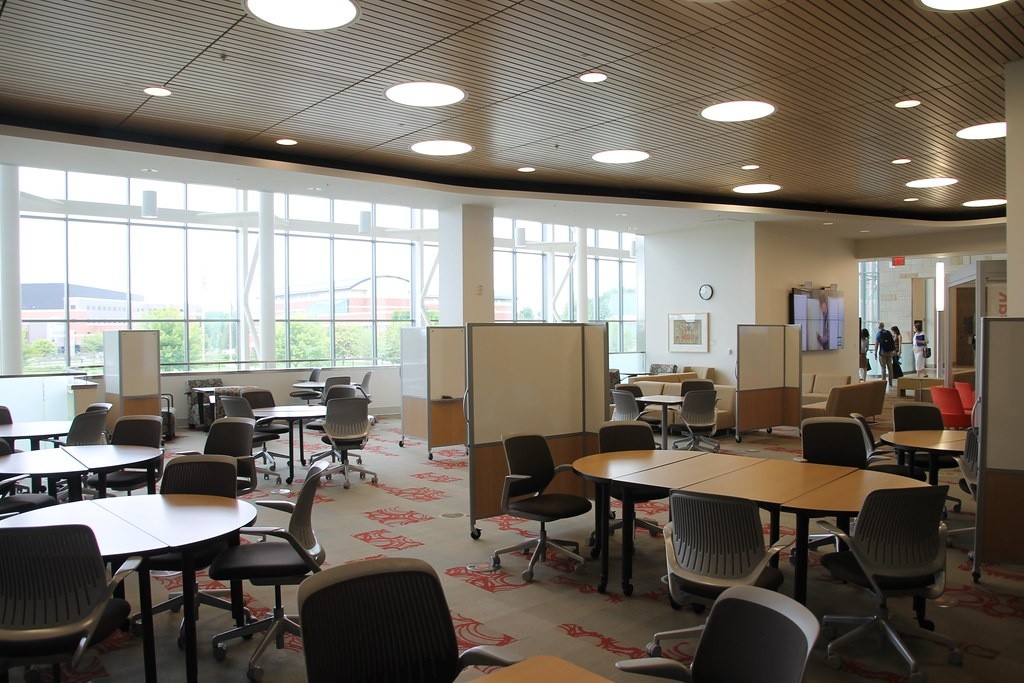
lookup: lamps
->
[359,211,371,234]
[630,240,635,257]
[514,227,526,247]
[143,190,158,220]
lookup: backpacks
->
[891,354,904,379]
[877,329,896,353]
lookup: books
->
[916,335,926,346]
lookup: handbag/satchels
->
[866,353,873,373]
[923,342,933,359]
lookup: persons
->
[874,322,893,385]
[859,328,869,381]
[816,303,830,349]
[913,324,928,377]
[891,326,903,379]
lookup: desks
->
[633,395,685,451]
[251,405,331,484]
[0,421,73,451]
[192,387,217,425]
[572,450,936,631]
[0,493,257,682]
[466,654,618,683]
[0,445,163,503]
[873,429,969,486]
[620,372,649,381]
[293,381,353,392]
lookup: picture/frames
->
[668,312,709,353]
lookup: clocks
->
[698,283,713,300]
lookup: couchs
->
[608,380,736,437]
[628,373,698,387]
[683,366,715,380]
[801,374,851,405]
[802,379,887,423]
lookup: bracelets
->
[875,354,877,355]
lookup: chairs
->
[297,558,528,683]
[491,369,977,682]
[0,367,379,682]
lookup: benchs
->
[649,363,677,373]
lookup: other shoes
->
[924,374,929,379]
